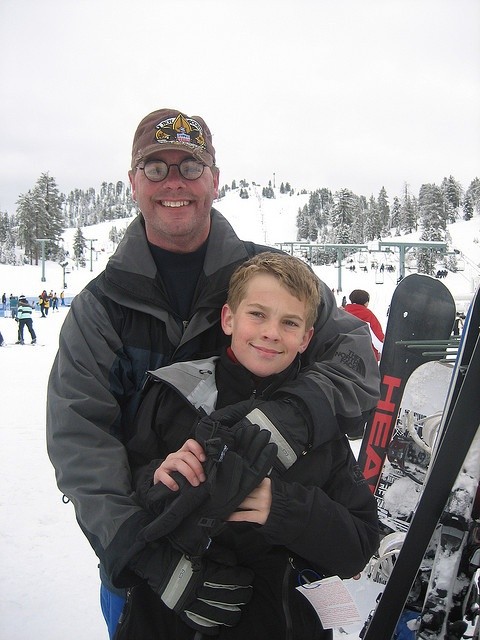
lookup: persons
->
[337,287,385,360]
[16,295,37,344]
[2,292,16,317]
[46,110,380,640]
[112,251,380,639]
[37,290,66,318]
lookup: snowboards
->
[356,273,456,492]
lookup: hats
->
[130,108,216,167]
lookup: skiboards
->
[430,282,479,477]
[417,475,480,640]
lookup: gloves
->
[137,420,241,541]
[165,424,278,556]
[207,399,311,478]
[132,541,255,636]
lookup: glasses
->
[135,160,204,181]
